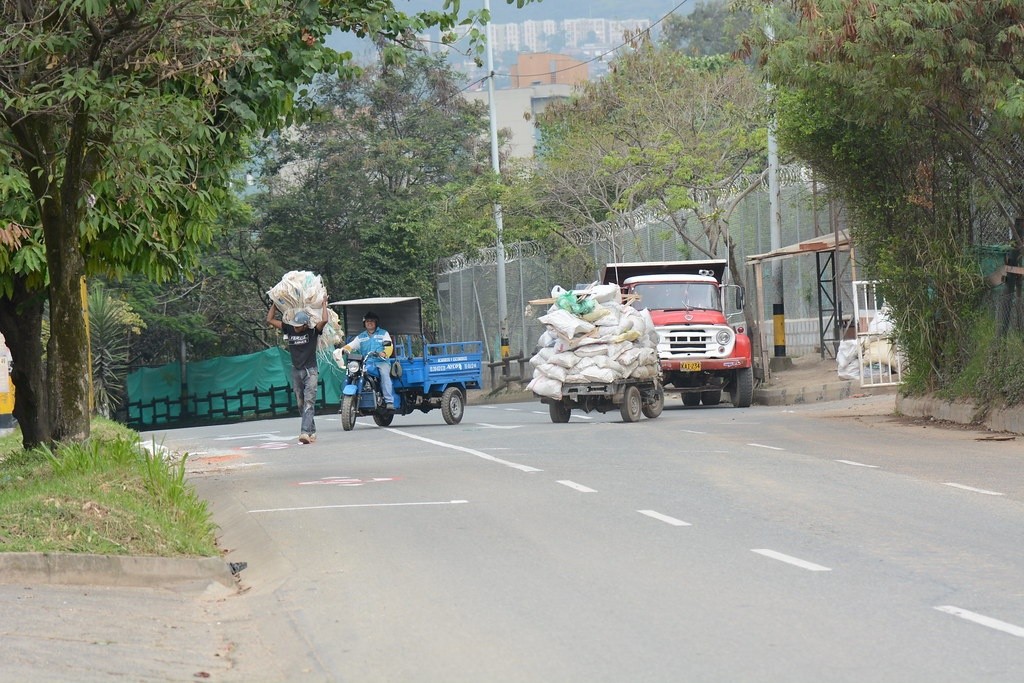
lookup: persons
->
[341,313,394,409]
[267,296,329,444]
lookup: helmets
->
[362,311,379,327]
[289,311,309,327]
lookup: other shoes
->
[386,402,394,410]
[310,432,317,442]
[299,434,311,444]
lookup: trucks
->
[601,258,754,408]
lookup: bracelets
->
[322,301,326,306]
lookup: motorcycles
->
[532,347,666,423]
[327,297,483,431]
